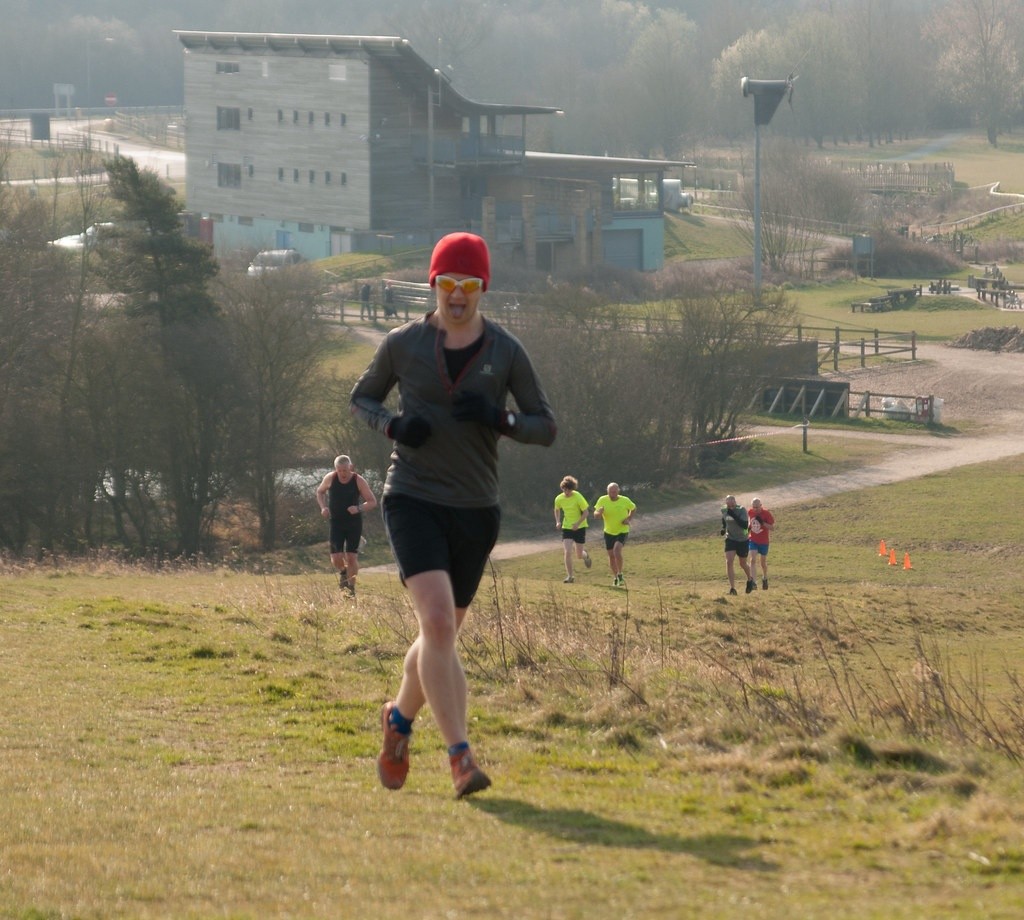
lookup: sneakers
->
[449,749,492,798]
[339,571,348,591]
[584,550,591,567]
[345,591,355,598]
[376,701,409,790]
[565,576,575,583]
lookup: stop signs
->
[105,92,119,107]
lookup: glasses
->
[434,274,483,292]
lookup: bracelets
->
[321,507,328,514]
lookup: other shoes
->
[613,578,619,586]
[753,583,758,590]
[763,579,769,589]
[620,579,626,586]
[747,578,753,594]
[729,589,737,595]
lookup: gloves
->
[755,516,763,525]
[727,509,734,517]
[453,394,502,432]
[720,528,725,536]
[387,415,430,448]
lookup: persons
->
[748,498,776,590]
[316,455,378,595]
[348,231,557,802]
[555,475,592,584]
[594,482,637,585]
[721,495,754,595]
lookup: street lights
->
[85,33,115,111]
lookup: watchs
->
[357,505,363,511]
[503,410,518,429]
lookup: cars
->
[248,248,306,278]
[48,220,112,255]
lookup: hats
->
[429,232,490,290]
